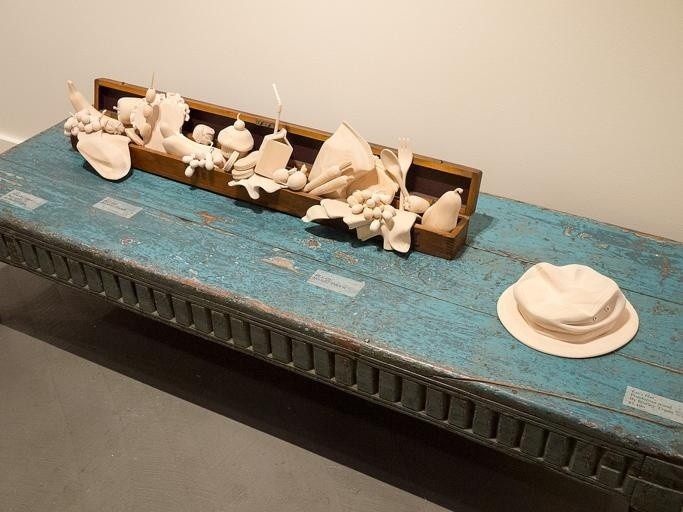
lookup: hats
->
[497,261,640,359]
